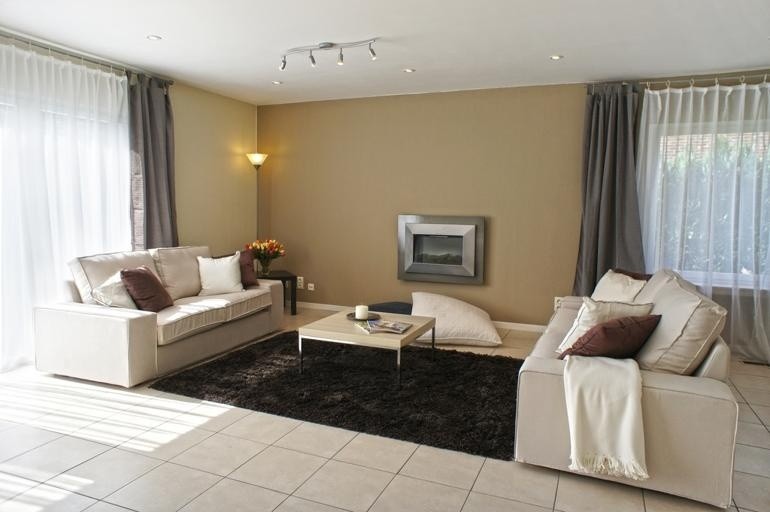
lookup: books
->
[355,319,413,333]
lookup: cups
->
[355,305,369,319]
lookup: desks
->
[257,270,297,314]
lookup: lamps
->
[247,153,270,242]
[279,43,376,71]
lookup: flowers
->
[245,239,287,271]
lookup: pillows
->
[121,265,174,311]
[153,245,212,300]
[555,296,654,357]
[633,269,702,306]
[614,267,653,281]
[91,269,139,310]
[196,251,246,297]
[639,277,729,373]
[591,269,646,303]
[69,249,165,306]
[211,249,260,286]
[411,291,503,346]
[557,315,662,359]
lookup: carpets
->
[151,331,525,463]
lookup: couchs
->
[33,246,285,389]
[515,268,738,509]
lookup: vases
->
[261,265,271,273]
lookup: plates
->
[346,312,380,321]
